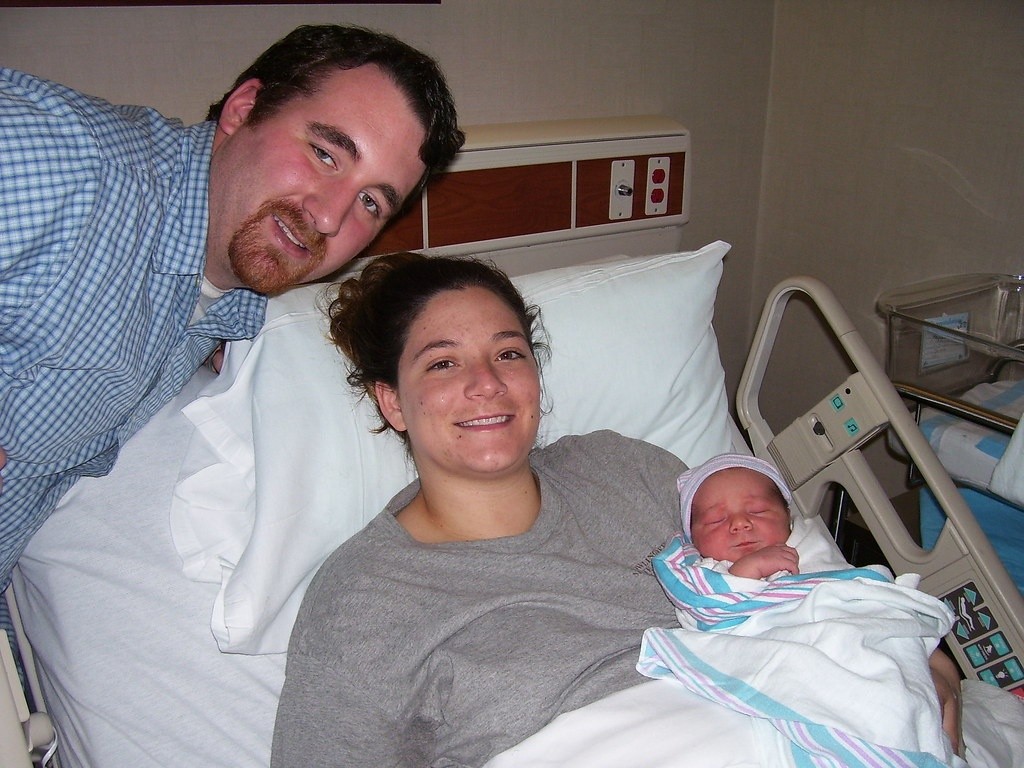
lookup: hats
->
[675,452,793,543]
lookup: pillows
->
[163,239,756,656]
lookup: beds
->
[0,242,1024,768]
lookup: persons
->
[676,453,800,580]
[0,26,465,688]
[269,250,966,767]
